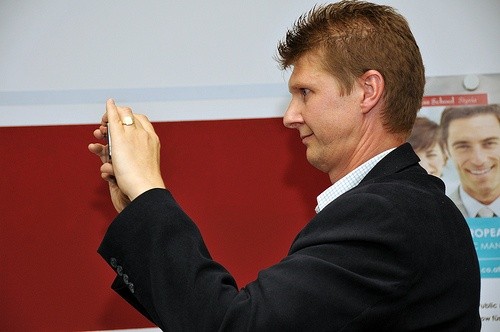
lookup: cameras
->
[106,122,115,180]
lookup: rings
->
[122,117,134,126]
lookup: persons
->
[408,117,449,179]
[87,1,483,332]
[439,105,500,219]
